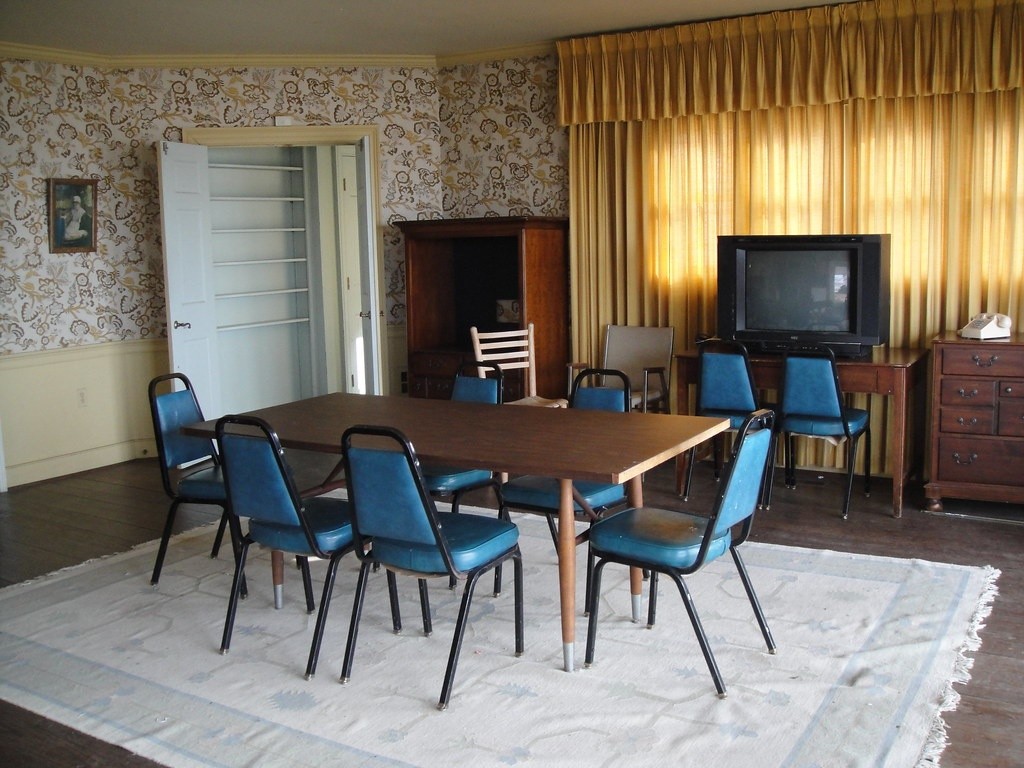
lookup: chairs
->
[493,366,631,619]
[470,318,570,487]
[217,413,404,685]
[339,423,526,712]
[602,324,676,483]
[684,339,791,510]
[581,407,779,698]
[766,340,871,520]
[417,360,505,589]
[146,372,305,601]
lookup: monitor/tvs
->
[718,234,883,361]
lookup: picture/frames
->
[48,178,97,255]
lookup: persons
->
[63,196,87,241]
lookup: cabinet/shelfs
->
[923,328,1024,518]
[392,216,573,405]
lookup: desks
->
[672,345,931,519]
[180,393,734,674]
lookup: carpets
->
[0,484,1004,768]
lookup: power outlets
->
[401,383,409,393]
[401,372,408,382]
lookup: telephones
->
[958,312,1016,341]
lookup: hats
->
[73,196,81,204]
